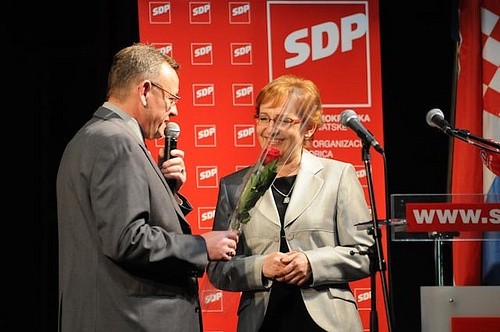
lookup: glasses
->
[251,111,305,130]
[152,82,181,106]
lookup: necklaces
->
[272,179,296,203]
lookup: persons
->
[57,43,238,332]
[206,74,379,332]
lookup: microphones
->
[340,110,384,153]
[426,108,455,137]
[164,122,180,189]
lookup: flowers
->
[222,89,319,260]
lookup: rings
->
[229,248,233,256]
